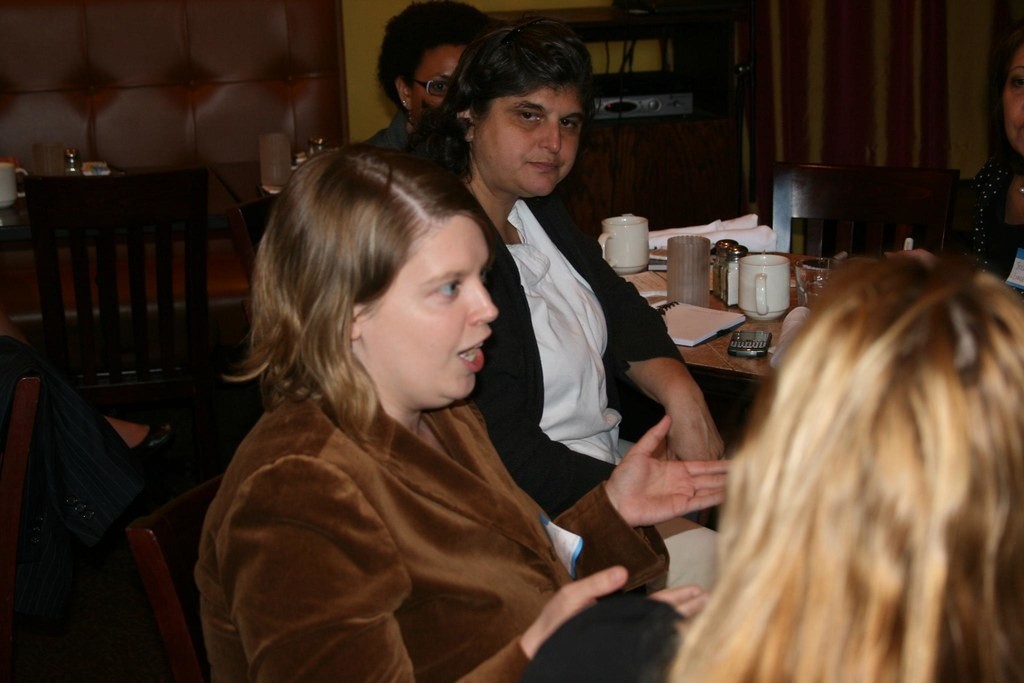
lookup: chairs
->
[771,159,961,259]
[1,165,223,683]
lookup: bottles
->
[61,147,82,177]
[307,134,327,158]
[714,238,752,307]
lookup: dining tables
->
[0,147,299,249]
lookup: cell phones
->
[727,330,772,357]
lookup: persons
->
[358,0,505,161]
[191,135,737,683]
[963,12,1024,300]
[410,10,725,601]
[512,245,1024,683]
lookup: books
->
[655,298,749,348]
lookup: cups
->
[793,256,848,310]
[598,216,650,276]
[258,132,292,194]
[738,254,791,321]
[33,140,63,177]
[0,163,30,209]
[665,235,710,309]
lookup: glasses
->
[414,78,450,96]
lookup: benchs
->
[0,0,344,364]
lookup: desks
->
[626,239,827,391]
